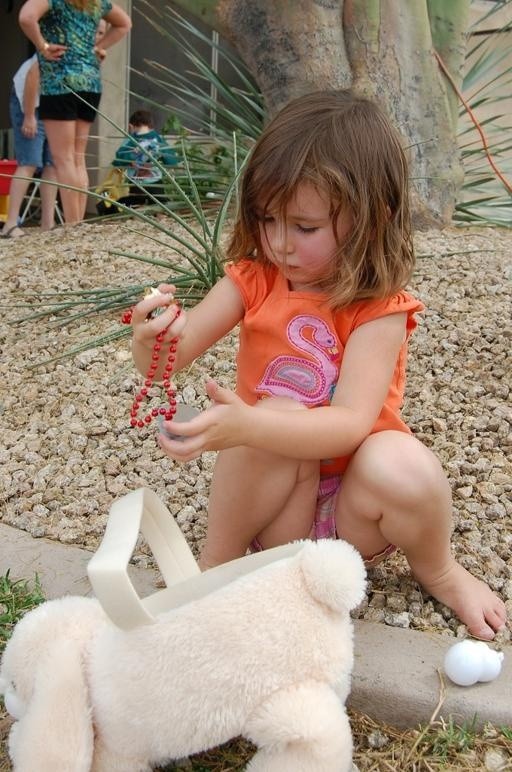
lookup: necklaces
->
[122,281,184,427]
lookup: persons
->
[112,108,178,207]
[128,90,509,640]
[19,0,133,221]
[0,16,107,240]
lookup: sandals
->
[0,226,30,243]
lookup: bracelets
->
[40,42,49,57]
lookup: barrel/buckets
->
[0,159,18,194]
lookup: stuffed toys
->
[94,165,129,216]
[1,537,369,772]
